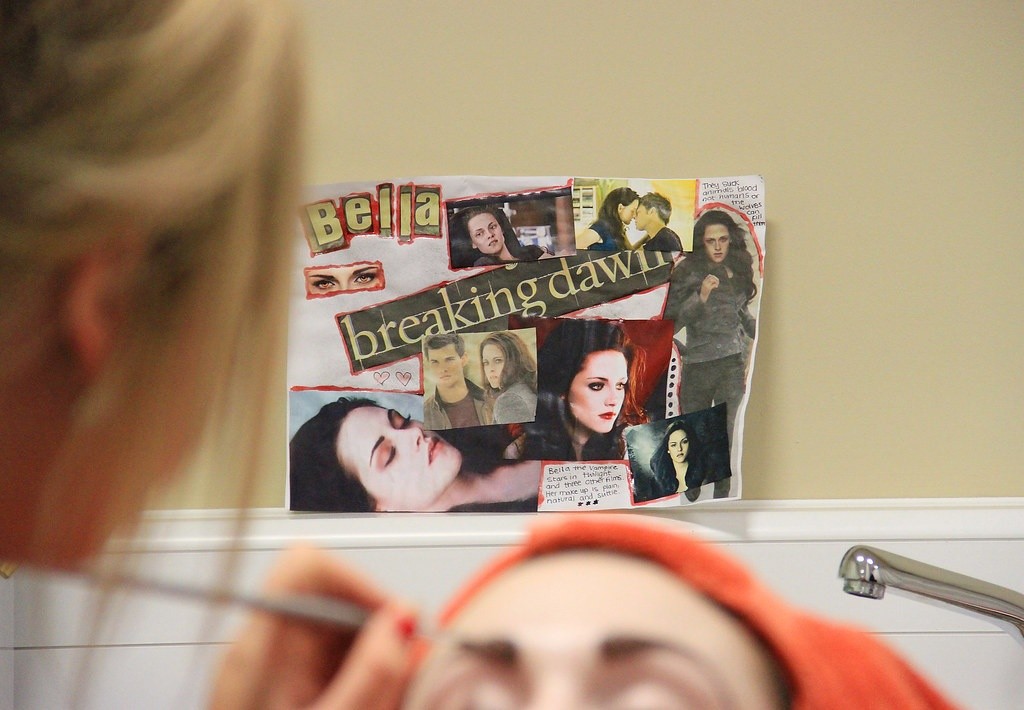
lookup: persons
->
[451,199,555,266]
[2,0,430,710]
[423,336,499,431]
[396,519,869,709]
[303,259,385,298]
[285,393,565,515]
[649,417,723,501]
[662,208,757,501]
[579,185,640,252]
[481,332,538,425]
[631,192,685,251]
[537,318,651,497]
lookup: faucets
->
[835,545,1024,643]
